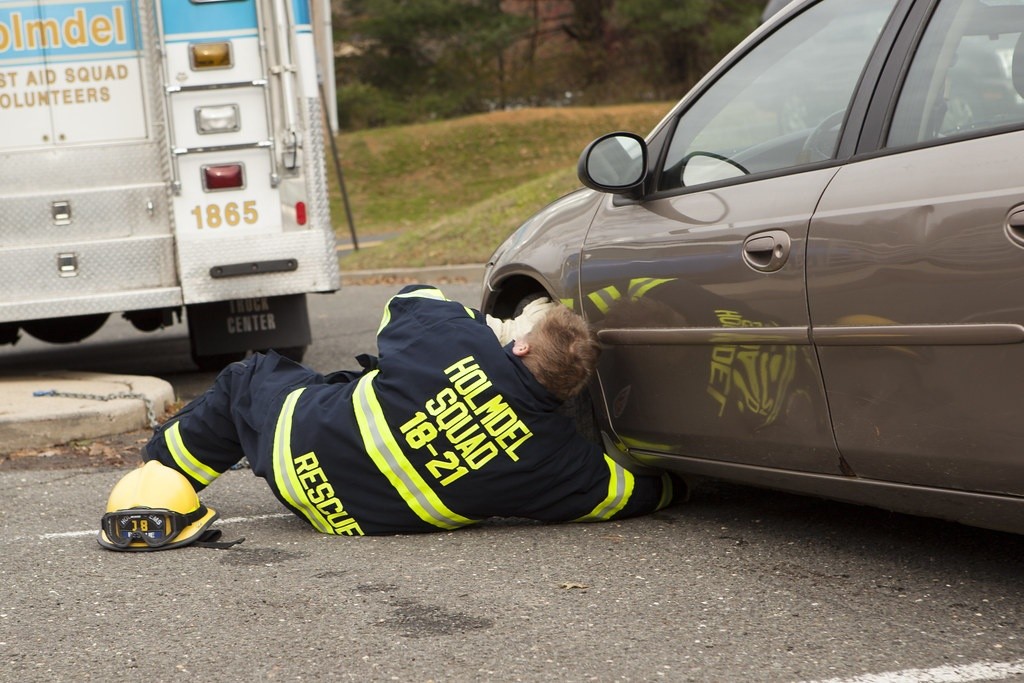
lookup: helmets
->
[96,460,220,552]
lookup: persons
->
[141,284,690,537]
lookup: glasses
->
[102,501,209,548]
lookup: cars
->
[483,0,1024,531]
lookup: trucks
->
[1,0,341,373]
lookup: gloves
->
[500,297,556,347]
